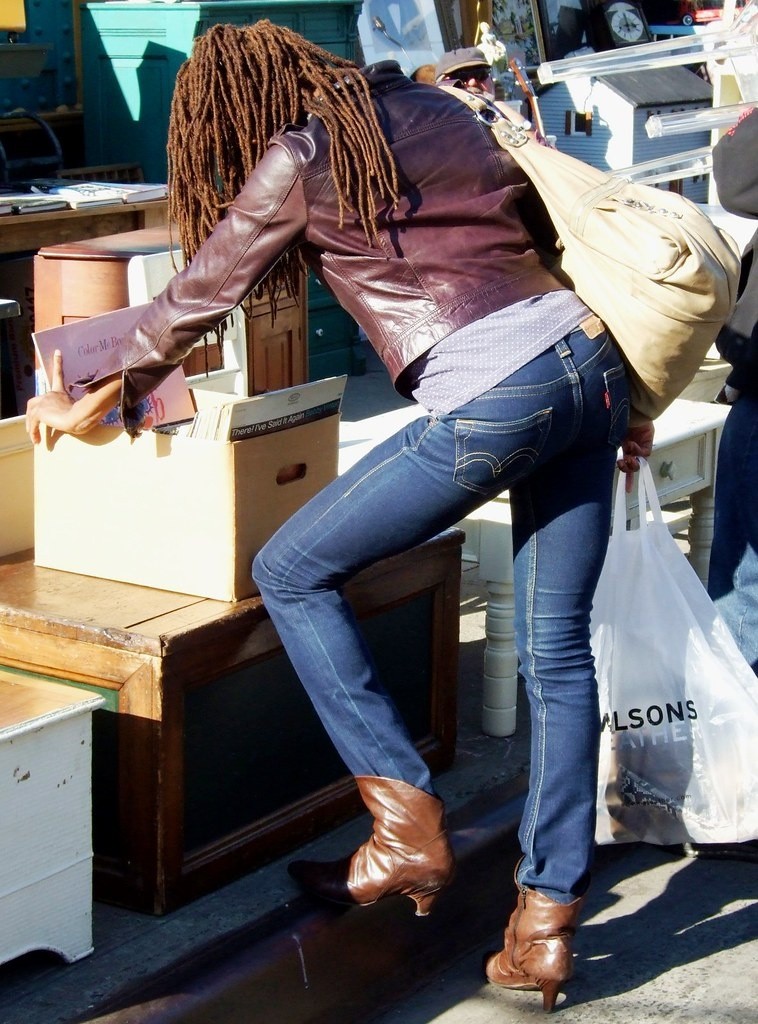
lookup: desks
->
[0,187,311,395]
[337,398,730,736]
[0,672,107,969]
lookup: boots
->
[482,855,592,1011]
[286,776,456,915]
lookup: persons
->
[685,103,758,857]
[25,20,653,1013]
[436,48,552,148]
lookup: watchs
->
[523,120,533,131]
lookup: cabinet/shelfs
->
[0,521,465,920]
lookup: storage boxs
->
[31,359,347,603]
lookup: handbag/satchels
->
[438,86,741,429]
[594,457,758,846]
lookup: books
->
[152,375,347,440]
[31,301,195,432]
[0,178,171,216]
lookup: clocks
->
[606,8,653,46]
